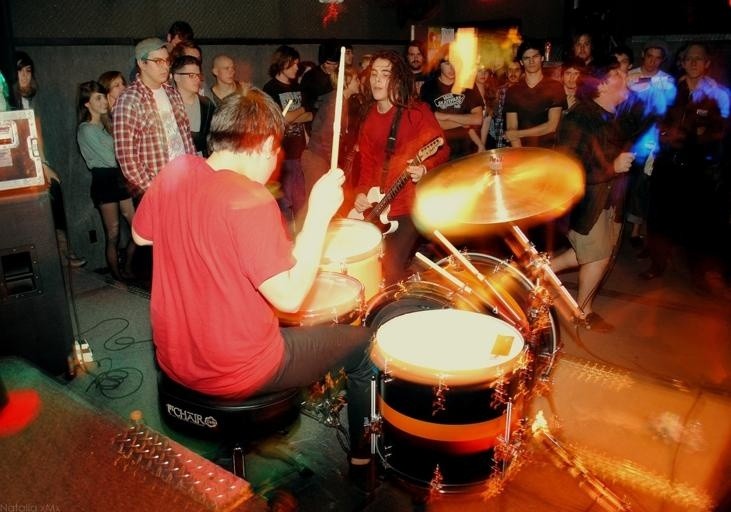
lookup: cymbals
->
[416,147,583,225]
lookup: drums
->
[319,218,386,305]
[369,310,528,493]
[365,253,557,399]
[276,271,366,399]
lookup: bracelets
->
[42,160,51,169]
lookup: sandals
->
[65,252,87,267]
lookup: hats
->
[642,37,669,51]
[134,38,173,60]
[319,41,340,62]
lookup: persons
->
[351,49,449,288]
[74,20,378,293]
[407,28,730,338]
[14,48,90,270]
[128,86,388,471]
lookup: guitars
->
[346,138,444,238]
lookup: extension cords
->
[75,339,93,362]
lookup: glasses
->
[176,72,203,80]
[146,58,173,66]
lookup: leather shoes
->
[0,387,42,438]
[573,312,616,334]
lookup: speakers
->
[0,187,75,377]
